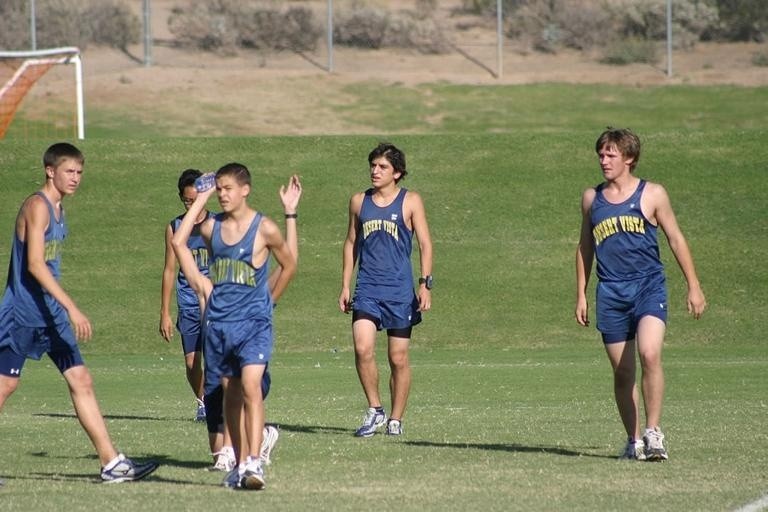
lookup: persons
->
[575,128,707,462]
[172,172,302,472]
[0,143,157,485]
[339,141,433,436]
[200,163,296,490]
[159,166,301,420]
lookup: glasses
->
[179,194,197,208]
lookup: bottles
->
[195,176,218,192]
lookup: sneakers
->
[355,407,387,437]
[642,427,668,461]
[222,463,245,490]
[385,418,402,436]
[618,436,647,460]
[194,396,206,423]
[101,454,159,484]
[259,426,279,464]
[208,446,236,472]
[241,456,265,490]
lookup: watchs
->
[281,213,296,218]
[415,276,432,291]
